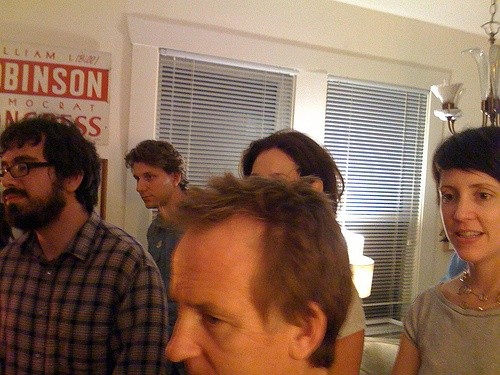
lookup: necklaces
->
[457,270,500,305]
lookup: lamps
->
[429,0,500,136]
[348,252,374,299]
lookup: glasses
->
[0,162,54,179]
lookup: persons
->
[239,128,365,375]
[158,173,354,375]
[391,125,500,375]
[125,139,190,375]
[0,112,168,375]
